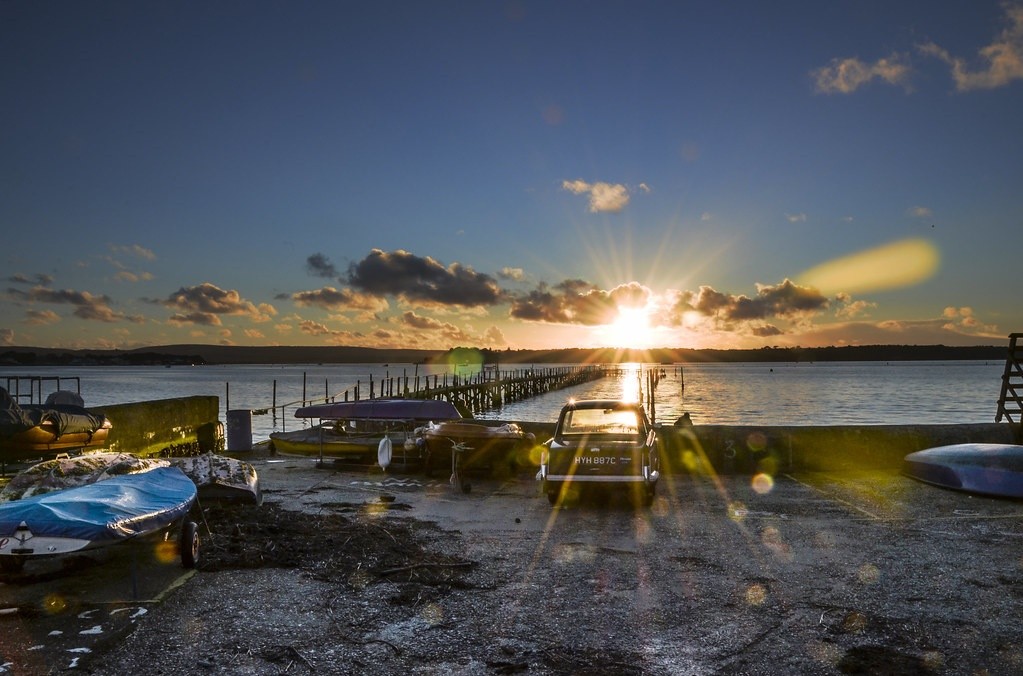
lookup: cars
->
[544,399,661,509]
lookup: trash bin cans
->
[225,409,253,452]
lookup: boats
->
[0,464,198,582]
[268,399,536,494]
[0,388,112,453]
[900,443,1023,499]
